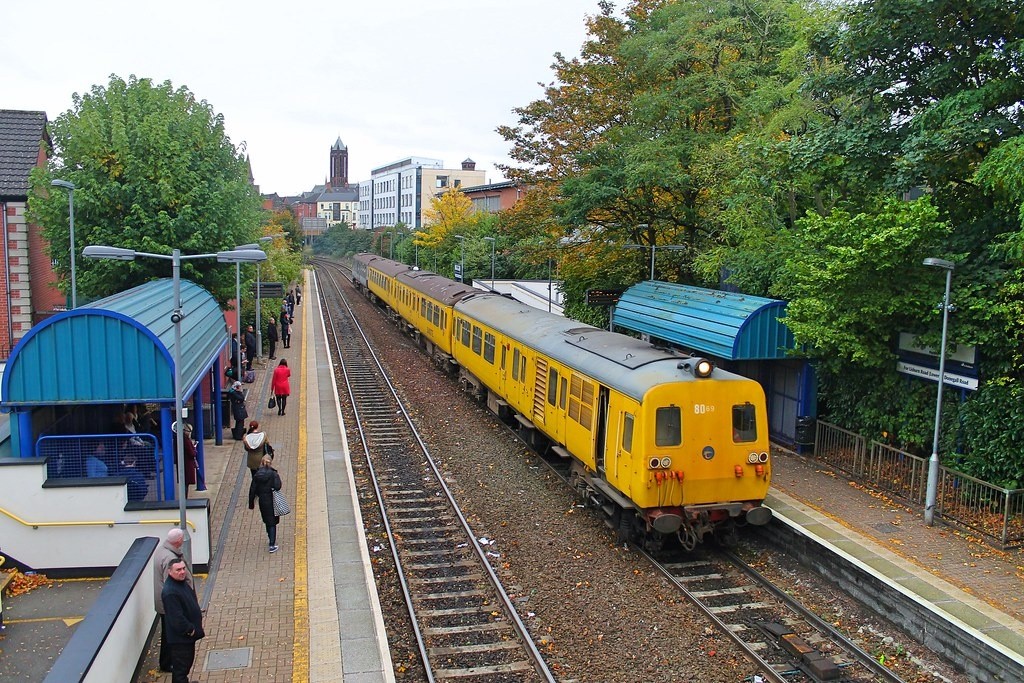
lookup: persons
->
[230,325,256,384]
[229,381,245,440]
[280,282,295,348]
[249,454,282,553]
[242,421,271,479]
[295,284,303,307]
[174,425,197,500]
[161,559,206,683]
[271,359,291,416]
[268,318,278,360]
[153,528,195,672]
[67,403,161,502]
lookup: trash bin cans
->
[212,389,232,428]
[794,415,815,445]
[188,404,214,439]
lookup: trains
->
[350,253,772,559]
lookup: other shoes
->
[284,346,289,349]
[282,411,286,416]
[162,665,173,673]
[236,438,242,441]
[247,368,254,371]
[271,356,277,360]
[297,304,301,307]
[269,546,279,552]
[278,409,282,416]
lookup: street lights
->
[236,244,260,382]
[256,237,273,364]
[378,232,382,257]
[50,178,76,310]
[922,256,954,526]
[624,244,686,343]
[82,245,269,593]
[484,236,495,292]
[397,233,403,264]
[412,233,417,266]
[539,241,551,313]
[455,235,465,283]
[387,232,392,259]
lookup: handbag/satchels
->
[263,443,274,460]
[225,368,233,377]
[288,325,292,334]
[297,293,301,296]
[268,397,276,408]
[273,489,290,516]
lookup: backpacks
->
[247,372,254,383]
